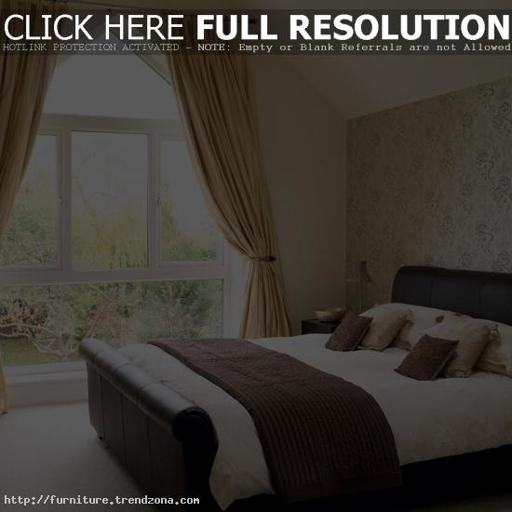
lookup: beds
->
[78,264,512,512]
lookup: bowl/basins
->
[315,307,346,321]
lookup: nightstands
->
[302,318,336,334]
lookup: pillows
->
[423,317,498,376]
[393,335,458,381]
[386,301,458,352]
[358,304,409,351]
[325,310,373,352]
[463,315,511,378]
